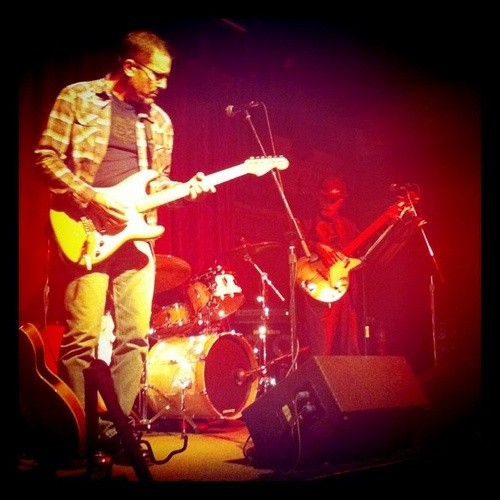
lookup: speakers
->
[243,356,433,471]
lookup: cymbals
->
[154,252,191,291]
[219,240,276,257]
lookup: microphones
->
[390,183,405,191]
[234,367,250,382]
[226,101,260,118]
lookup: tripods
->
[137,362,199,435]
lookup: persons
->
[34,32,218,468]
[296,177,395,356]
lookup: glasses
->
[134,60,170,82]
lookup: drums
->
[151,302,193,339]
[186,265,246,321]
[144,330,259,421]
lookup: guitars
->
[294,201,408,304]
[49,154,289,269]
[18,322,86,470]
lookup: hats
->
[318,175,347,204]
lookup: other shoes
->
[107,436,153,468]
